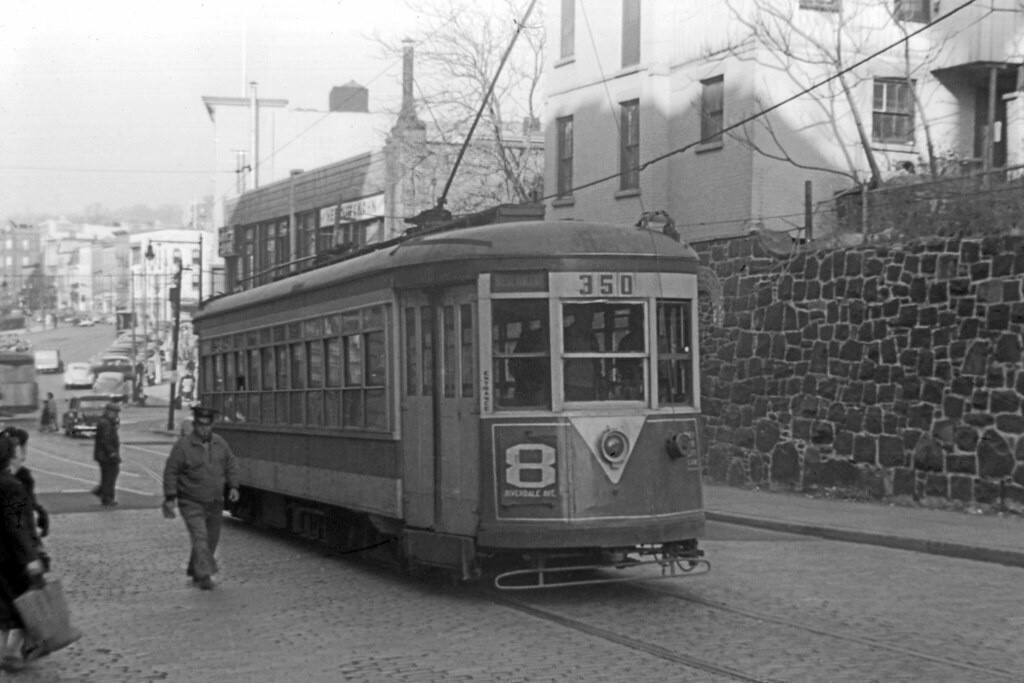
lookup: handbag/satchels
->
[13,574,81,661]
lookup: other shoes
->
[201,574,212,589]
[102,499,118,505]
[187,569,199,582]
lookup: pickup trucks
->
[118,308,156,334]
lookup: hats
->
[191,406,219,424]
[106,403,121,412]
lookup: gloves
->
[111,454,121,464]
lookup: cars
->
[35,350,65,375]
[63,396,121,436]
[63,328,169,387]
[0,296,117,330]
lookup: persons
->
[90,404,122,506]
[163,406,239,590]
[39,393,60,433]
[508,308,603,400]
[616,308,644,400]
[0,428,52,672]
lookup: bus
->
[192,1,711,590]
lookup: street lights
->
[145,232,203,308]
